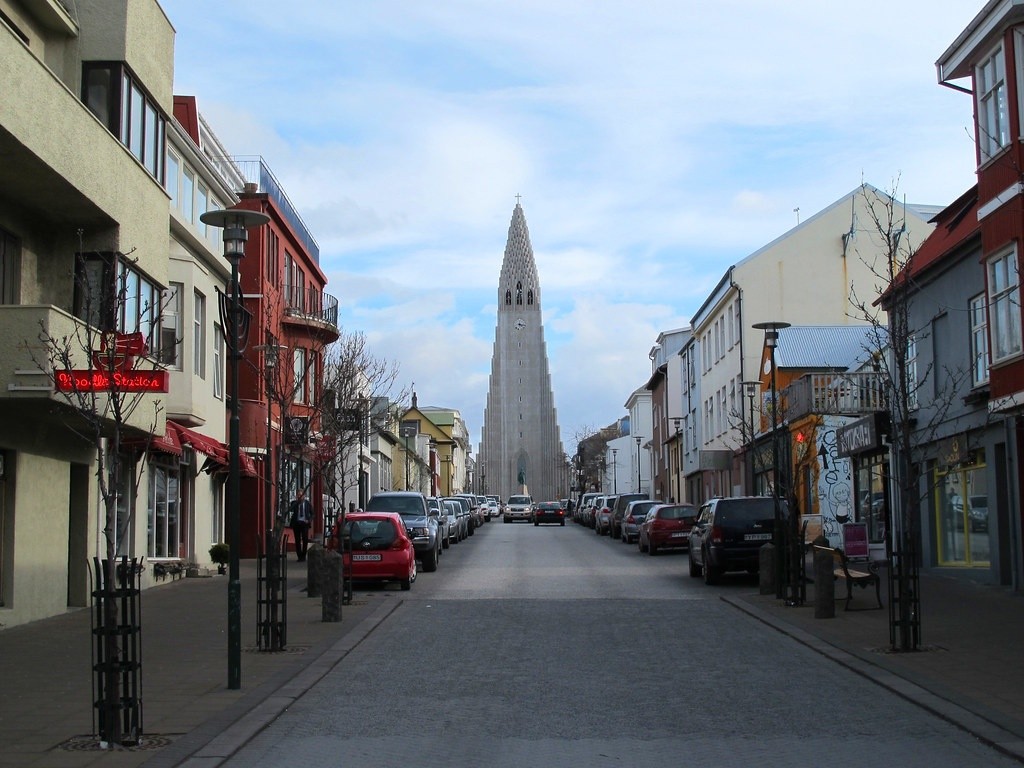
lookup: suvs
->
[502,494,536,523]
[683,495,792,585]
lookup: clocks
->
[514,319,526,330]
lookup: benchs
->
[810,544,884,612]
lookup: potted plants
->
[209,542,230,575]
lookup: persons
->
[288,490,314,562]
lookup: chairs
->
[346,522,361,540]
[376,522,395,540]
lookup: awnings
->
[114,419,258,480]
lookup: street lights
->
[399,427,416,491]
[600,456,608,494]
[200,209,271,691]
[633,436,646,494]
[426,443,437,497]
[609,449,620,494]
[442,454,452,497]
[346,398,373,513]
[668,417,686,503]
[738,380,764,495]
[752,322,792,602]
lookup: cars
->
[572,493,700,556]
[327,491,507,591]
[532,501,566,526]
